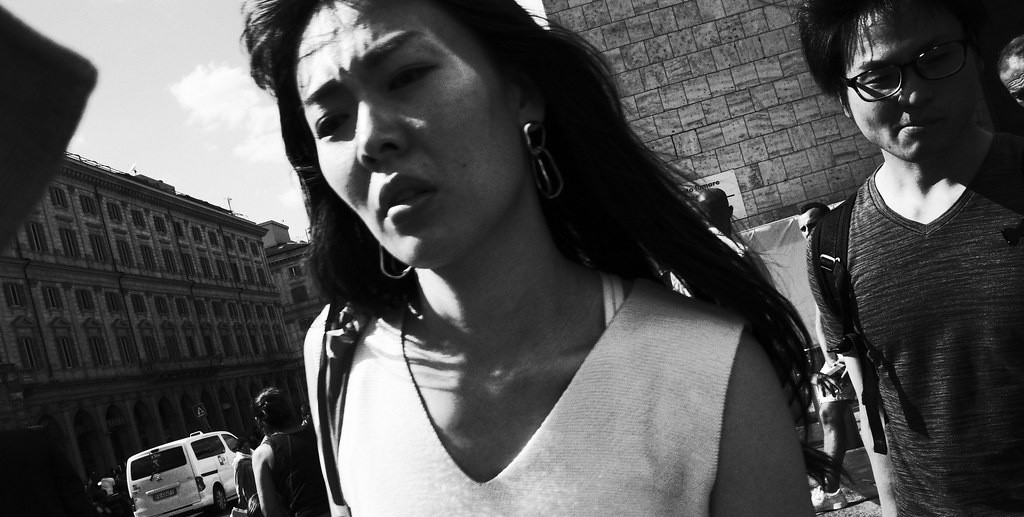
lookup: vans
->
[125,430,256,516]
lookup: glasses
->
[839,35,978,102]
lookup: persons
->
[239,387,332,517]
[100,464,125,496]
[240,0,857,517]
[669,0,1024,517]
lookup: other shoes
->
[810,485,848,512]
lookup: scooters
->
[84,464,135,517]
